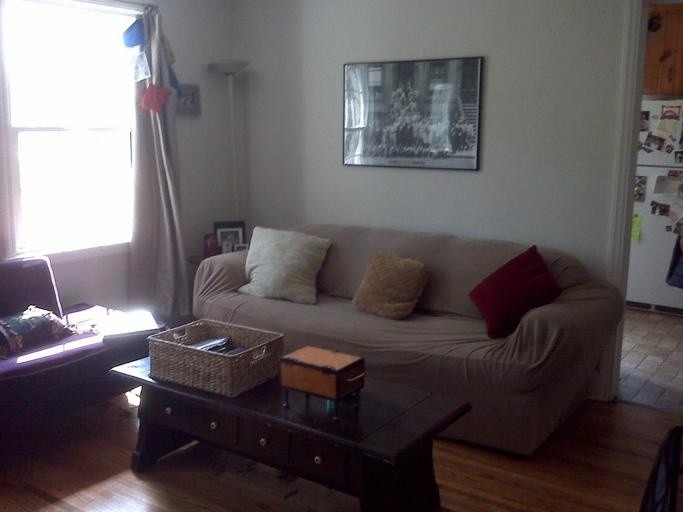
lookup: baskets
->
[149,318,285,399]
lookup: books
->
[191,335,246,356]
[94,311,159,341]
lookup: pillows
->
[469,245,560,334]
[239,225,330,306]
[352,250,427,322]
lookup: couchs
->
[0,257,164,407]
[194,224,622,455]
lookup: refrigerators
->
[626,93,683,315]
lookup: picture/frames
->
[214,222,244,251]
[342,57,484,171]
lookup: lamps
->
[210,60,253,224]
